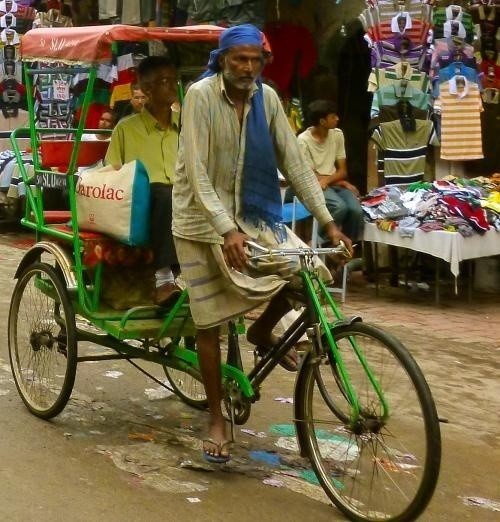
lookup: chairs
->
[36,134,156,271]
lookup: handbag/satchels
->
[67,158,151,248]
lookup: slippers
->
[277,336,302,372]
[203,437,232,464]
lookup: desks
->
[362,215,499,305]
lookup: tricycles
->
[6,19,443,522]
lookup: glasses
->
[147,76,182,89]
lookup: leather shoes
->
[153,282,182,305]
